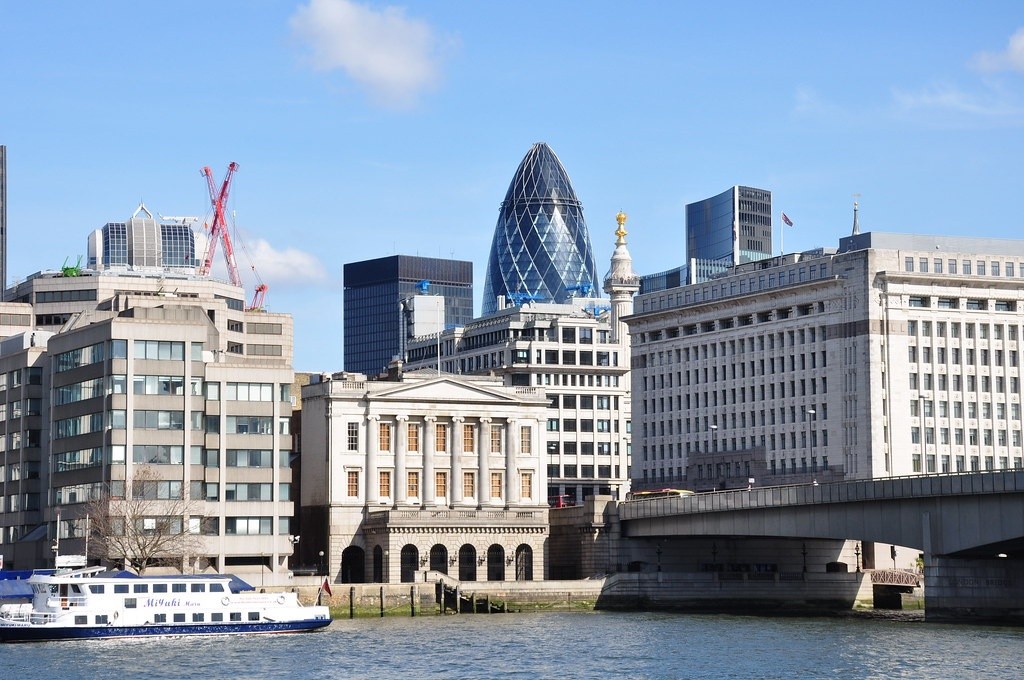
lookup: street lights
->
[384,551,389,584]
[319,551,324,575]
[801,542,809,574]
[655,544,662,572]
[712,541,717,567]
[855,544,861,573]
[805,409,816,482]
[708,425,718,491]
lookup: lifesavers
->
[114,611,119,620]
[277,596,286,604]
[221,596,230,606]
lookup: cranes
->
[584,306,611,315]
[565,282,594,296]
[507,289,547,306]
[414,279,431,295]
[194,161,267,312]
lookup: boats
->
[1,565,332,641]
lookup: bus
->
[549,494,575,508]
[626,491,695,501]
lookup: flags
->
[322,579,332,597]
[782,212,793,227]
